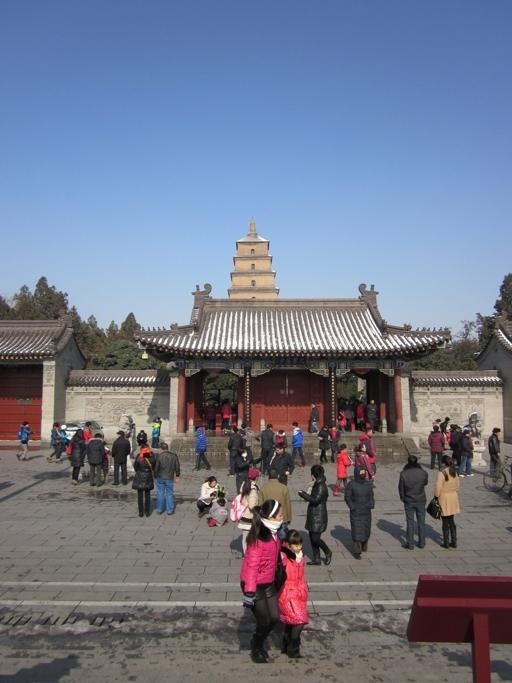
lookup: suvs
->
[59,418,104,442]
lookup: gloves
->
[242,593,258,609]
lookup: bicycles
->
[477,455,512,492]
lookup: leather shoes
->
[323,552,334,566]
[307,559,322,566]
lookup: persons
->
[193,428,211,471]
[60,425,68,452]
[428,426,446,471]
[205,400,217,432]
[318,425,330,462]
[366,399,376,433]
[47,422,62,463]
[488,428,502,479]
[102,441,109,482]
[112,431,130,485]
[399,455,429,550]
[355,443,376,481]
[241,423,253,464]
[458,430,473,477]
[276,527,309,658]
[151,417,161,449]
[154,443,180,515]
[345,465,374,559]
[271,444,294,486]
[239,430,246,448]
[433,419,441,427]
[234,449,252,494]
[87,433,106,487]
[238,469,261,560]
[197,476,229,528]
[329,426,341,462]
[275,429,288,450]
[221,399,231,426]
[82,422,93,466]
[360,428,376,475]
[137,430,147,445]
[310,402,319,433]
[258,469,292,525]
[333,444,354,496]
[298,464,332,565]
[17,421,35,460]
[450,424,461,466]
[255,424,275,476]
[435,455,460,548]
[291,422,306,467]
[227,427,243,477]
[338,397,364,432]
[441,417,450,434]
[70,429,85,485]
[132,444,156,517]
[239,499,283,663]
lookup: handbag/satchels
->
[427,496,442,521]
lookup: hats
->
[116,431,126,435]
[248,470,260,479]
[493,428,501,433]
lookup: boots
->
[277,634,290,654]
[288,637,304,658]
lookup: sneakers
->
[111,481,120,486]
[97,480,105,487]
[123,482,127,485]
[158,510,163,514]
[458,475,465,478]
[365,546,370,552]
[450,542,459,547]
[402,544,414,551]
[167,512,174,515]
[467,473,470,478]
[198,511,206,518]
[89,483,96,486]
[442,544,450,550]
[252,649,265,663]
[72,479,81,486]
[420,544,426,549]
[471,474,474,477]
[46,456,53,463]
[16,454,20,461]
[354,552,363,560]
[55,459,62,463]
[259,645,271,659]
[23,458,30,461]
[139,512,144,518]
[334,491,342,497]
[193,467,198,471]
[204,467,210,470]
[146,512,150,517]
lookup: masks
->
[361,474,367,479]
